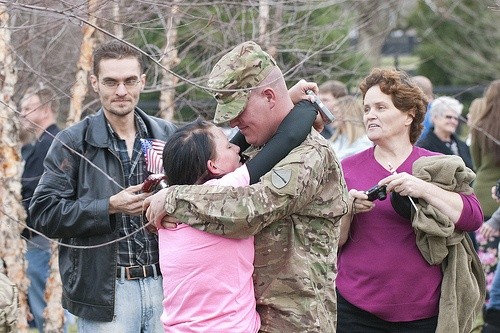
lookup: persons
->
[157,79,324,333]
[28,38,180,333]
[142,40,349,333]
[17,86,69,333]
[319,67,500,333]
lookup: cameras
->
[142,174,169,195]
[363,184,387,202]
[306,89,336,126]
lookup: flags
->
[140,138,167,173]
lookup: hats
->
[207,39,277,123]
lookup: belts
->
[116,262,161,280]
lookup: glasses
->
[97,76,141,88]
